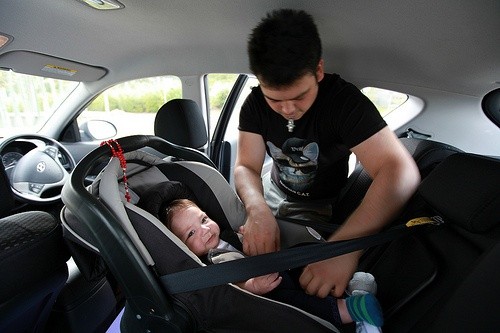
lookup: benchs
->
[323,137,500,332]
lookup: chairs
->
[58,148,352,333]
[0,209,74,333]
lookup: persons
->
[231,5,424,298]
[162,198,386,330]
